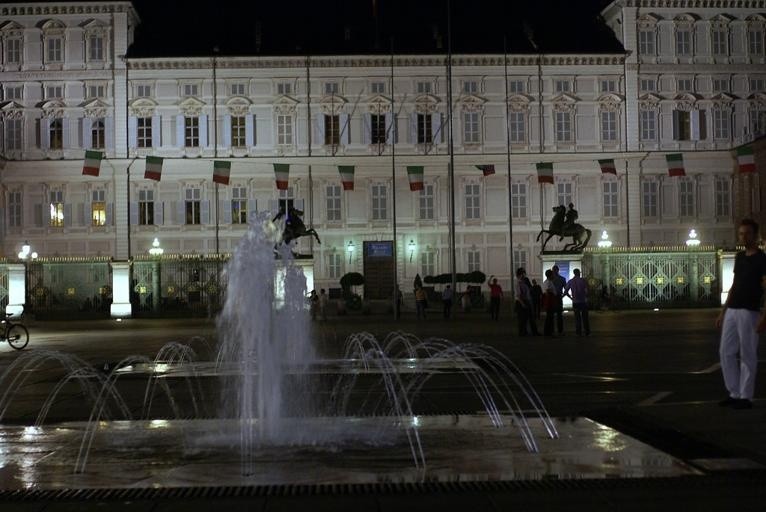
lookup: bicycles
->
[0,312,29,349]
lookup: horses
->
[536,205,593,253]
[274,206,321,260]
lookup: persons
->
[272,198,304,223]
[442,284,453,321]
[514,264,610,337]
[714,217,766,410]
[414,283,427,320]
[318,288,328,322]
[391,284,404,320]
[487,274,506,322]
[457,284,474,311]
[558,203,579,241]
[309,290,318,321]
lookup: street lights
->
[18,241,38,313]
[684,228,701,304]
[597,228,613,300]
[148,238,164,313]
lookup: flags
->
[665,153,687,177]
[81,150,103,176]
[337,164,356,191]
[273,164,290,191]
[475,164,496,177]
[144,156,163,182]
[735,144,756,174]
[212,160,231,185]
[405,165,427,192]
[597,158,617,175]
[535,162,556,184]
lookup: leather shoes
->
[720,397,749,410]
